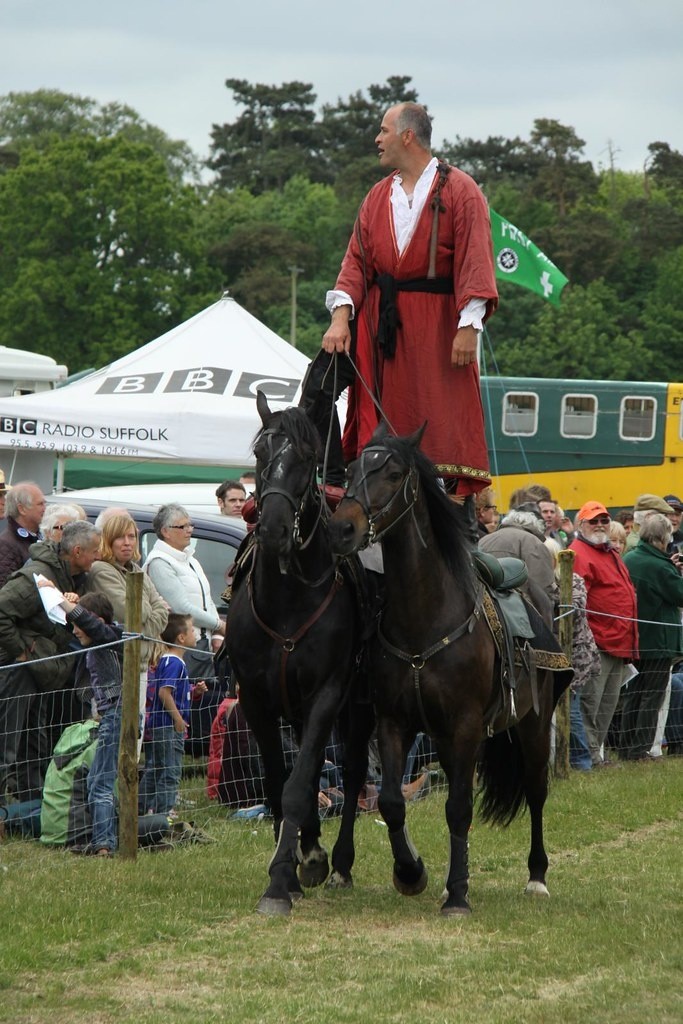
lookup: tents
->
[0,292,351,467]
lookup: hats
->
[577,501,610,520]
[558,507,569,520]
[663,495,683,511]
[633,494,675,513]
[0,470,13,492]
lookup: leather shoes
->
[402,772,428,801]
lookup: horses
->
[224,390,555,918]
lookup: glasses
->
[581,519,610,525]
[52,523,65,530]
[168,522,196,531]
[486,504,497,512]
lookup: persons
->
[0,470,683,856]
[297,100,499,543]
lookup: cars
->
[39,483,262,639]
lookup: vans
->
[475,376,683,528]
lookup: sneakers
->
[175,797,197,810]
[167,817,174,826]
[167,821,219,845]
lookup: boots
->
[450,490,480,549]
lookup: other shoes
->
[169,810,178,819]
[662,739,668,748]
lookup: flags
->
[489,204,570,304]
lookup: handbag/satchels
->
[182,638,218,689]
